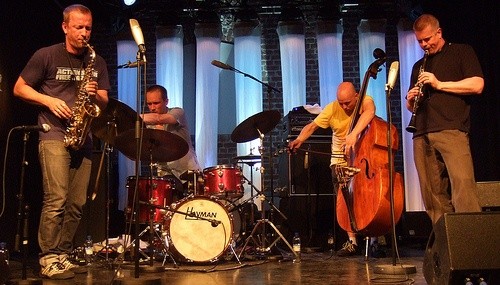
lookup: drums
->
[123,175,184,225]
[200,164,244,200]
[165,195,243,266]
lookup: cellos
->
[332,48,405,260]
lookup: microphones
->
[210,59,230,69]
[129,18,145,54]
[385,60,401,93]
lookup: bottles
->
[0,242,9,266]
[292,232,301,263]
[84,236,94,267]
[117,234,125,262]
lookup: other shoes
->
[337,240,359,256]
[369,240,386,257]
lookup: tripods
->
[4,133,38,269]
[87,57,301,269]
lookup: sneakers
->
[59,256,88,274]
[39,262,75,279]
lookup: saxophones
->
[63,39,101,153]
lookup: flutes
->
[406,48,430,133]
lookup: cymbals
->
[91,96,146,148]
[116,126,190,164]
[230,109,284,145]
[226,154,271,167]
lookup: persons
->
[405,14,485,228]
[13,5,111,280]
[139,85,203,184]
[286,81,387,257]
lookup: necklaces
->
[62,42,85,89]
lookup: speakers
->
[276,141,344,248]
[420,211,500,285]
[14,123,51,133]
